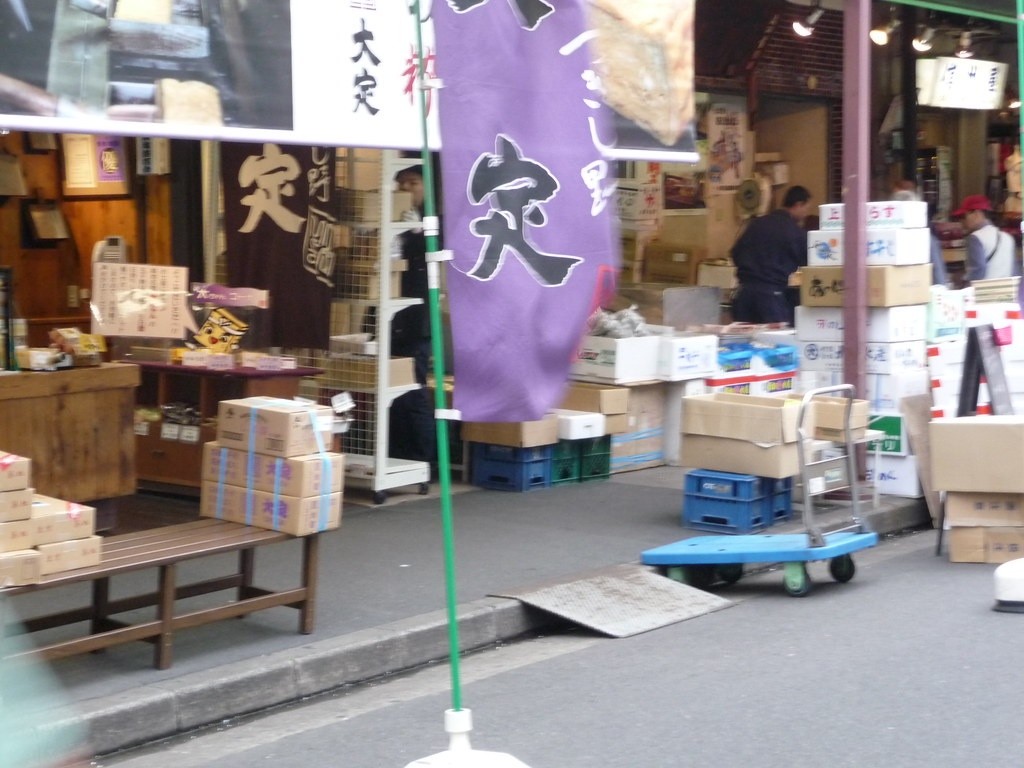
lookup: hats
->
[950,194,994,216]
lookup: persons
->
[394,165,429,382]
[948,196,1021,291]
[730,185,812,322]
[888,175,946,287]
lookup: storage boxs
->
[334,353,416,390]
[0,450,42,587]
[31,492,95,546]
[35,534,102,576]
[202,441,345,499]
[216,396,334,459]
[341,191,413,223]
[338,260,407,301]
[427,104,1024,561]
[199,480,344,537]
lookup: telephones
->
[90,236,130,266]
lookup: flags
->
[431,0,619,424]
[218,139,335,352]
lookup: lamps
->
[1005,85,1021,108]
[793,7,827,37]
[912,11,939,51]
[869,5,901,45]
[954,17,976,57]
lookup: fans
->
[736,173,772,218]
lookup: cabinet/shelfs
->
[111,148,430,499]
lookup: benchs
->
[0,519,319,676]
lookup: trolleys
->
[639,384,878,597]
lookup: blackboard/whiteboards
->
[955,324,1013,416]
